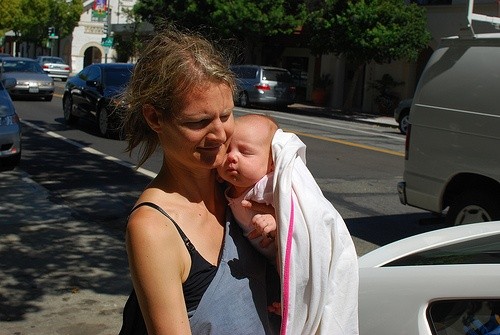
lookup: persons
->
[115,29,358,335]
[215,115,359,317]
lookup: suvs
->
[396,0,500,228]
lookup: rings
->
[267,234,275,242]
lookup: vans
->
[230,64,292,108]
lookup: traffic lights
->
[48,27,55,36]
[104,23,108,34]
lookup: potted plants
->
[309,73,335,107]
[375,73,405,114]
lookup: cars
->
[37,56,70,82]
[393,99,413,135]
[359,220,500,335]
[61,62,136,139]
[0,77,22,163]
[0,56,54,102]
